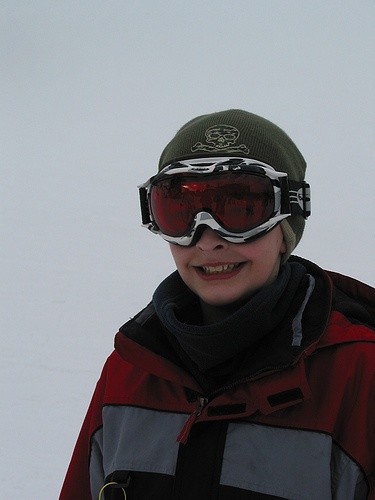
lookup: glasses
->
[138,166,291,247]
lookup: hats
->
[157,107,311,267]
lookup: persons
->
[59,110,375,500]
